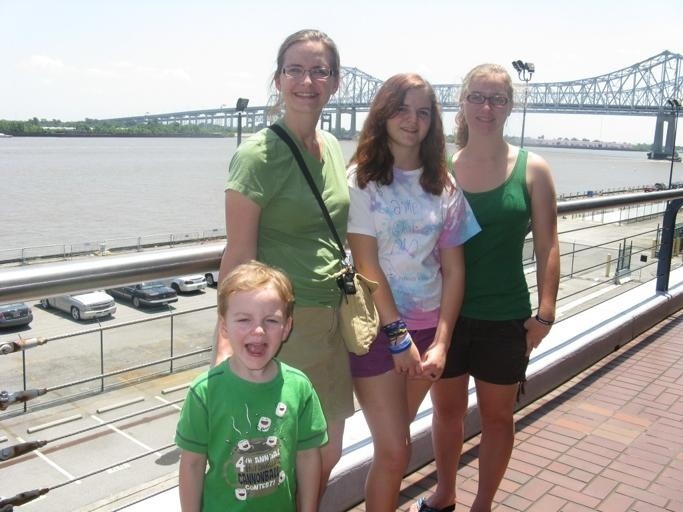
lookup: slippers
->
[411,497,456,512]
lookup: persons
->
[212,30,355,511]
[175,260,328,512]
[405,63,561,511]
[345,74,484,512]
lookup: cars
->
[1,270,220,329]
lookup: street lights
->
[512,60,535,148]
[668,98,681,191]
[236,98,249,147]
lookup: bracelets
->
[535,316,555,325]
[382,320,412,354]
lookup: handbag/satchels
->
[338,272,382,355]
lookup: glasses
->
[281,66,334,80]
[466,93,510,106]
